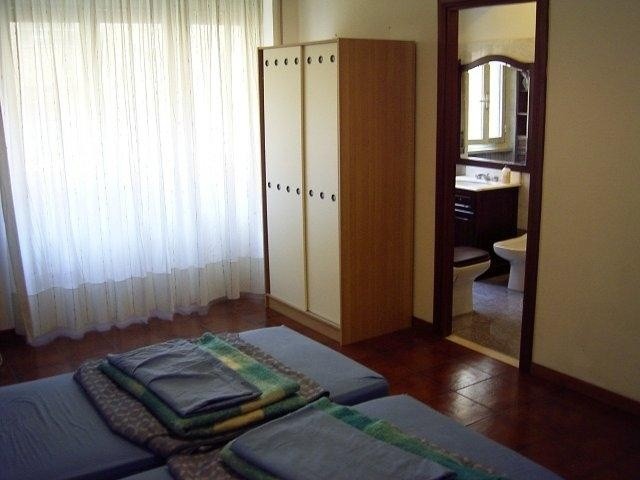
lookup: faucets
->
[477,174,490,181]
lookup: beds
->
[136,393,559,479]
[1,325,393,478]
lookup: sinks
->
[493,233,527,292]
[455,180,486,187]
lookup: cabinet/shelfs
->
[454,188,519,281]
[257,37,416,347]
[513,70,530,166]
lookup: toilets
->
[452,247,491,318]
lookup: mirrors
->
[457,54,534,173]
